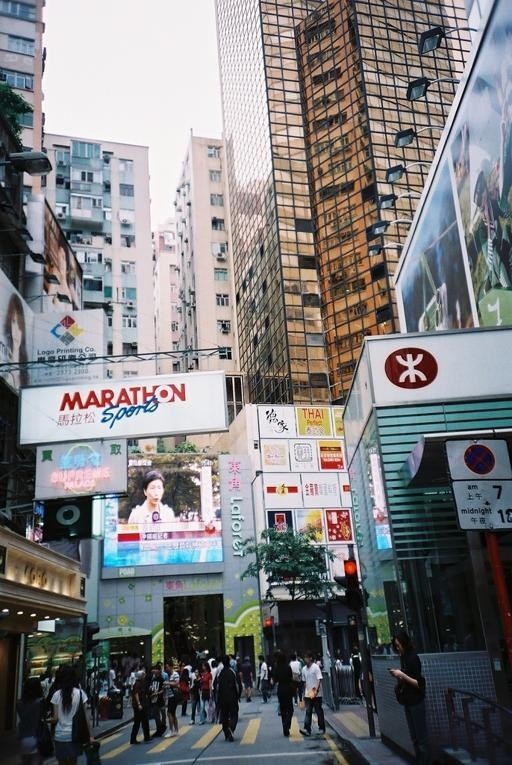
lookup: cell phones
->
[387,668,390,670]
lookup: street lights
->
[249,469,272,678]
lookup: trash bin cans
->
[108,688,123,719]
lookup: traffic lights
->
[333,572,350,608]
[312,602,330,628]
[263,617,274,640]
[343,557,365,610]
[83,626,102,652]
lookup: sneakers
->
[315,729,326,736]
[225,727,235,742]
[300,728,311,737]
[189,721,206,725]
[245,696,272,704]
[129,725,180,745]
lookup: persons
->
[389,633,432,765]
[128,471,175,523]
[4,293,30,393]
[42,647,365,764]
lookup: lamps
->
[368,24,479,257]
[0,152,71,304]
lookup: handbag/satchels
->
[71,688,91,744]
[18,737,40,756]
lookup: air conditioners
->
[122,218,131,225]
[128,300,135,307]
[216,252,225,260]
